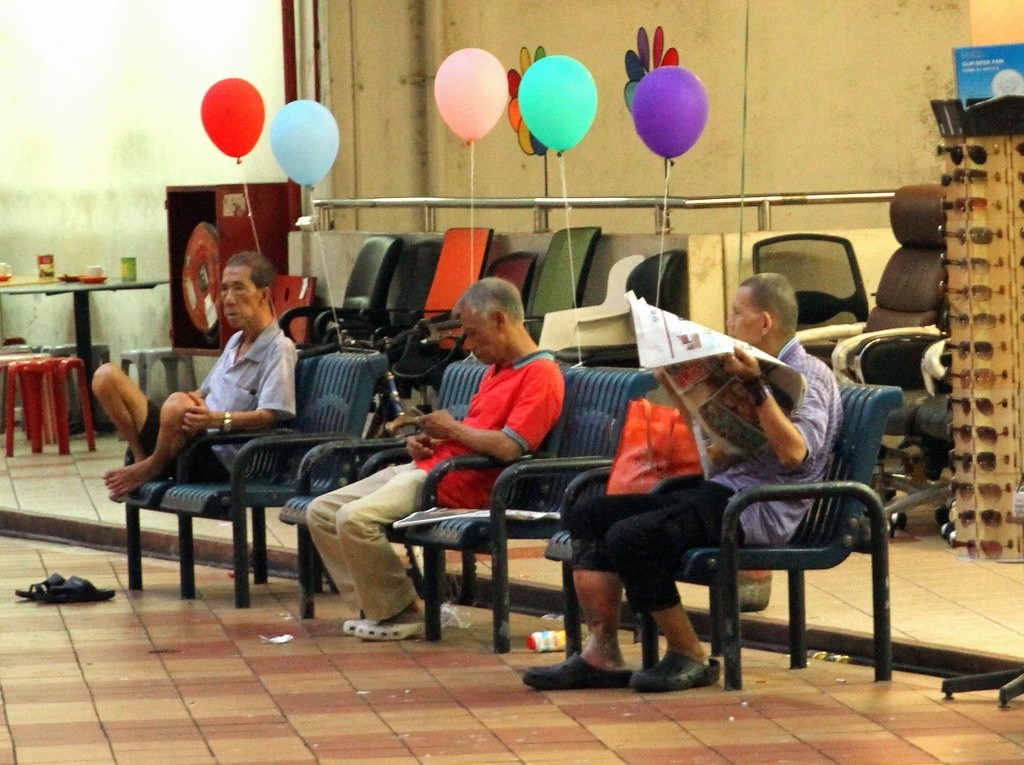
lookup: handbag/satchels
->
[606,398,703,496]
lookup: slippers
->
[629,651,722,692]
[342,618,365,635]
[522,652,632,692]
[36,575,116,604]
[355,611,428,642]
[15,573,66,600]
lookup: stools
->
[120,347,197,397]
[0,342,111,456]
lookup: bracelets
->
[223,412,231,431]
[741,373,773,406]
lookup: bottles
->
[121,258,136,282]
[39,256,53,280]
[527,631,566,652]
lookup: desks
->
[0,277,171,440]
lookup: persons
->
[91,250,298,500]
[522,271,844,694]
[305,276,565,641]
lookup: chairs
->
[112,354,317,592]
[159,353,388,608]
[794,180,956,547]
[639,383,906,693]
[278,182,870,369]
[278,360,490,620]
[394,366,662,653]
[544,464,616,662]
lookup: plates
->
[57,277,83,282]
[80,277,109,283]
[0,274,12,281]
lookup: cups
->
[0,263,12,276]
[86,267,102,277]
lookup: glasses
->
[937,136,1024,562]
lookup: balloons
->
[200,78,265,163]
[434,48,509,146]
[632,65,709,165]
[518,55,597,157]
[269,100,341,188]
